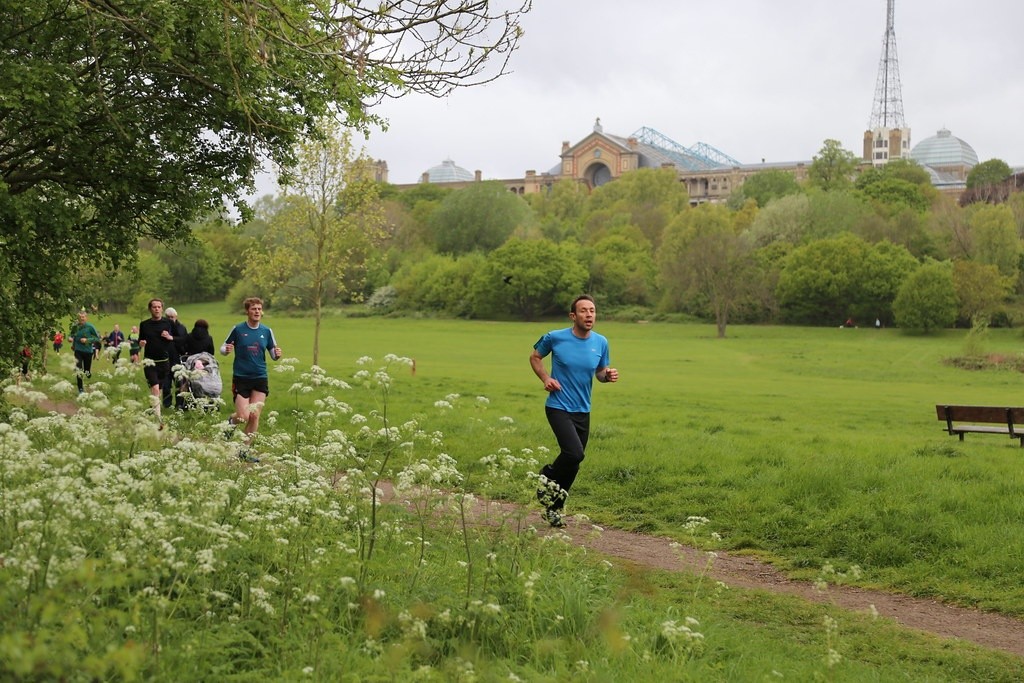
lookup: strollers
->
[176,352,223,411]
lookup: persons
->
[530,294,619,528]
[20,347,32,374]
[109,324,124,368]
[68,311,100,393]
[188,319,215,356]
[92,331,109,361]
[847,318,852,327]
[220,297,281,463]
[138,298,184,430]
[163,307,188,409]
[875,318,880,328]
[53,331,63,352]
[127,326,141,363]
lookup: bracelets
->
[605,376,609,382]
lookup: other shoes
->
[79,387,83,393]
[239,450,260,462]
[541,508,567,528]
[222,417,238,439]
[537,463,553,505]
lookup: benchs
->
[935,405,1024,446]
